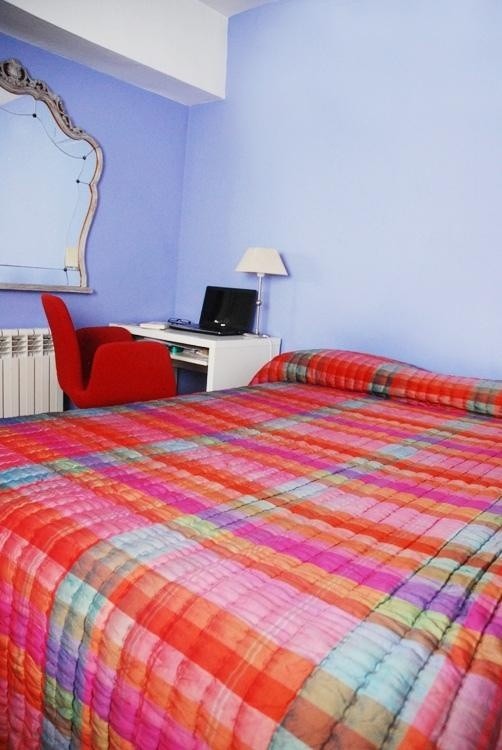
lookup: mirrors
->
[0,58,104,295]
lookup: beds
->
[0,349,502,746]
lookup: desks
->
[109,321,282,392]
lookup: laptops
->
[169,286,256,336]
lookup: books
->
[196,350,208,358]
[139,320,171,330]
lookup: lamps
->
[235,247,290,337]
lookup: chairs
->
[40,291,178,409]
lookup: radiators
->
[0,326,65,423]
